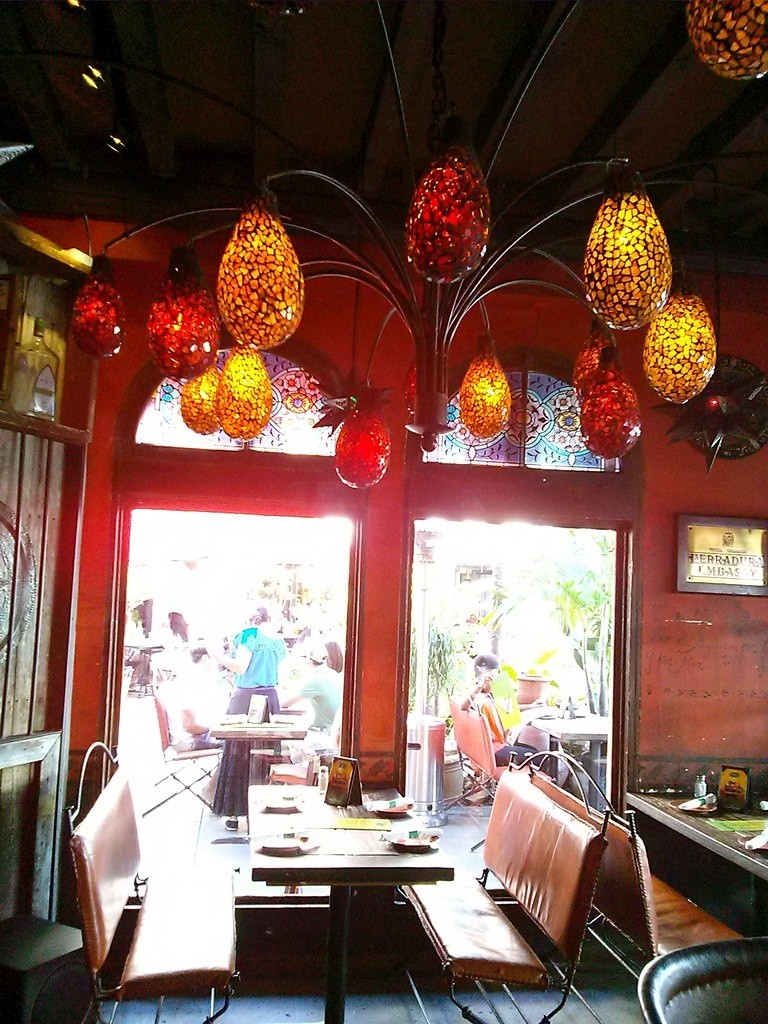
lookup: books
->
[734,830,761,837]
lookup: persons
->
[460,652,549,772]
[123,611,345,839]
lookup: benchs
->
[400,750,744,1024]
[61,742,237,1024]
[141,696,223,818]
[442,683,510,852]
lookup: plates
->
[389,834,439,849]
[375,804,413,819]
[738,838,768,850]
[261,844,299,857]
[669,799,717,814]
[264,802,298,812]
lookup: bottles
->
[694,774,707,797]
[318,767,329,796]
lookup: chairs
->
[638,938,768,1024]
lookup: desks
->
[527,711,609,812]
[625,792,768,938]
[248,784,455,1024]
[209,726,307,785]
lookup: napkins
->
[365,797,415,812]
[745,828,768,851]
[678,793,717,810]
[379,828,444,843]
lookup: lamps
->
[1,0,768,489]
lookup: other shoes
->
[225,819,239,831]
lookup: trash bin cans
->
[405,715,448,827]
